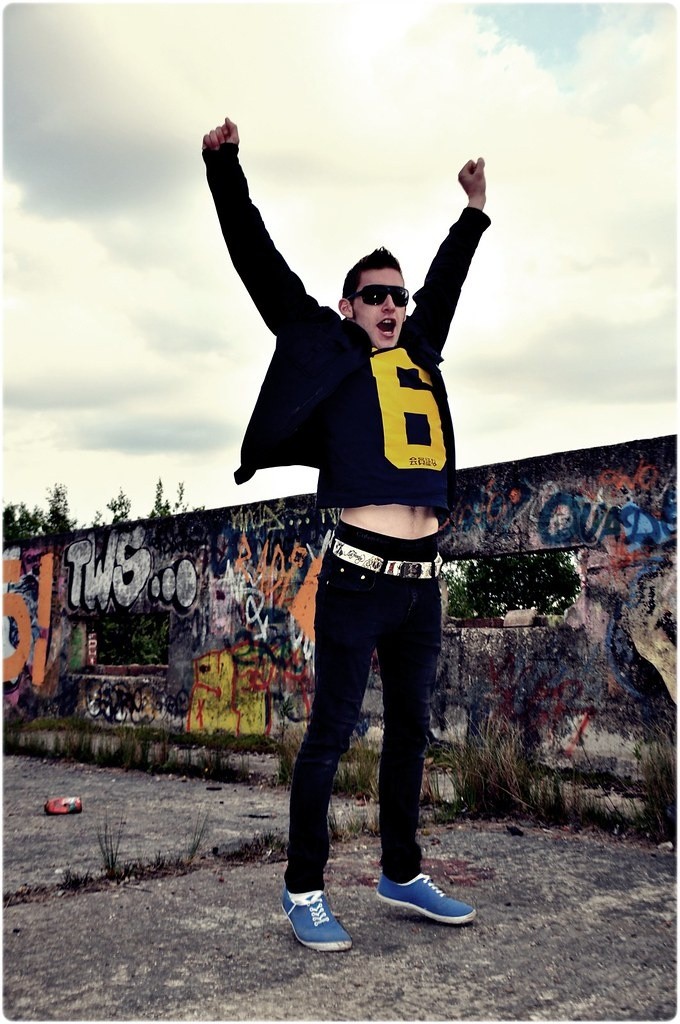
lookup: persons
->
[202,116,493,953]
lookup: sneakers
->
[375,869,479,927]
[280,885,354,952]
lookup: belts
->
[328,540,449,582]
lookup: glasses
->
[346,284,411,307]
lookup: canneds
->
[45,796,83,815]
